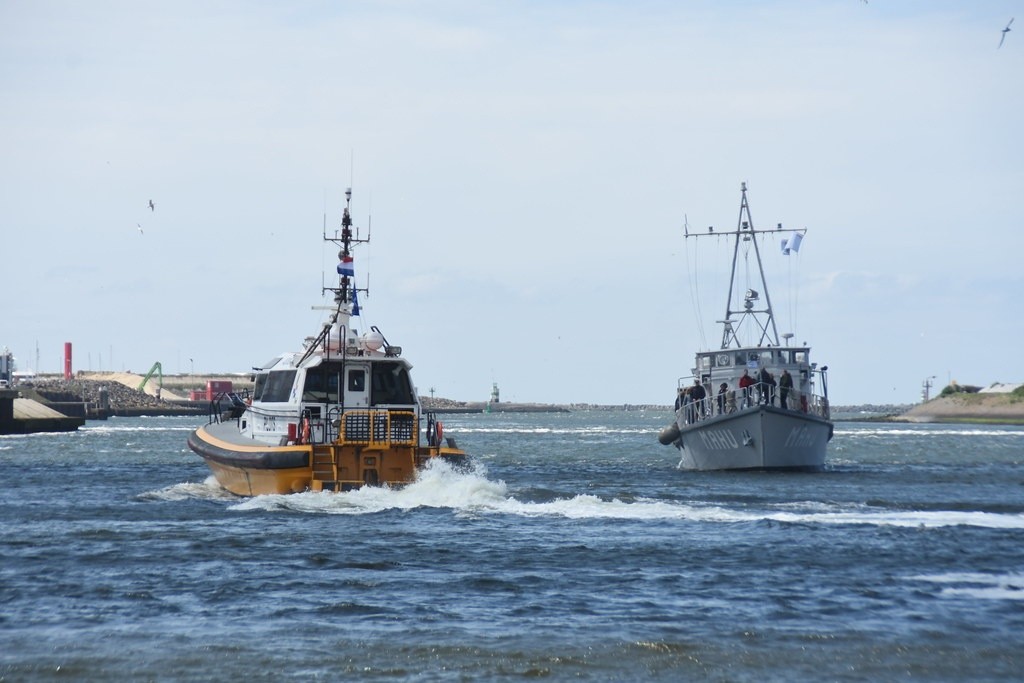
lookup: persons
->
[234,394,242,415]
[802,373,813,387]
[751,375,757,384]
[757,367,769,406]
[770,374,774,382]
[691,380,706,418]
[735,353,746,365]
[778,369,793,410]
[751,352,758,361]
[738,369,756,409]
[674,386,690,424]
[717,383,728,414]
[769,372,777,406]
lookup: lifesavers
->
[299,417,309,444]
[433,422,443,446]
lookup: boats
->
[661,182,834,470]
[188,189,466,499]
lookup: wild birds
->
[137,223,143,235]
[147,199,157,212]
[998,17,1014,50]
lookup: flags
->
[350,283,359,316]
[336,256,354,276]
[784,231,803,254]
[781,238,790,255]
